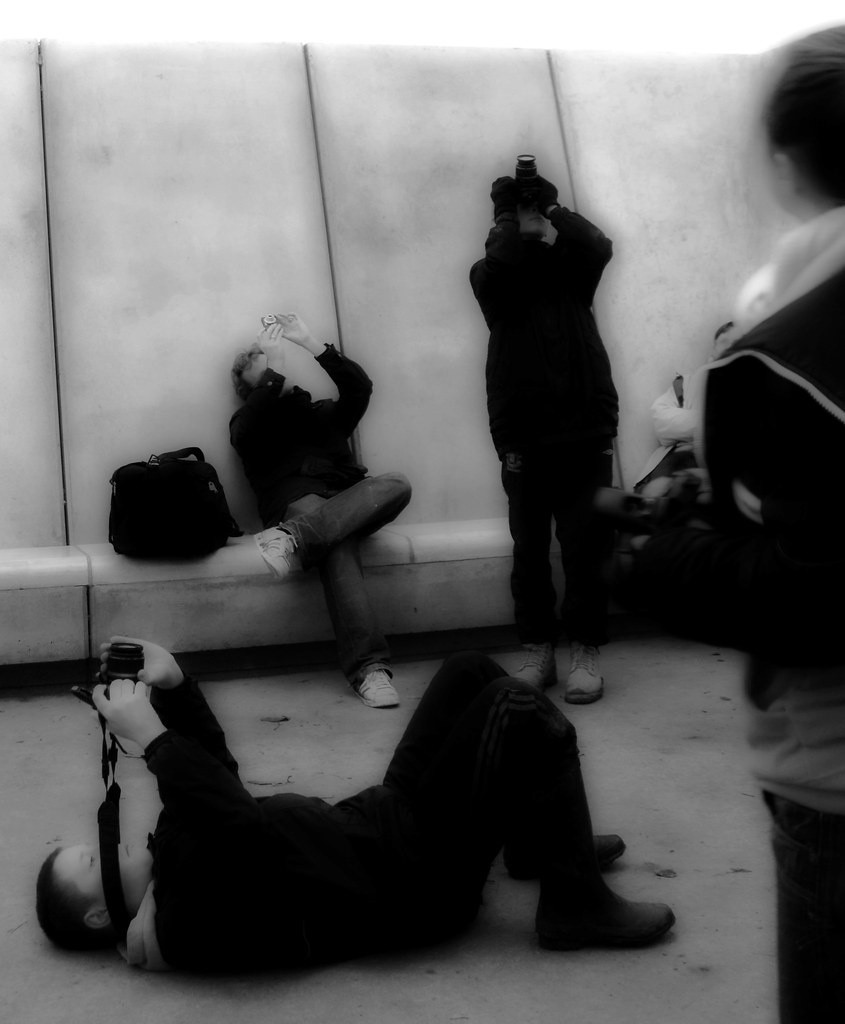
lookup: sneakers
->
[509,642,558,690]
[254,525,298,581]
[566,645,603,704]
[351,669,401,708]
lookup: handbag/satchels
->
[108,447,245,562]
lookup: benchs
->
[1,523,571,667]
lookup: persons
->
[589,321,736,574]
[628,27,845,1024]
[37,636,676,979]
[228,313,412,707]
[467,156,620,706]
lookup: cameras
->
[71,641,144,710]
[513,154,544,208]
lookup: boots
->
[503,825,625,881]
[525,757,677,952]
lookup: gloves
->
[528,175,560,220]
[490,176,518,220]
